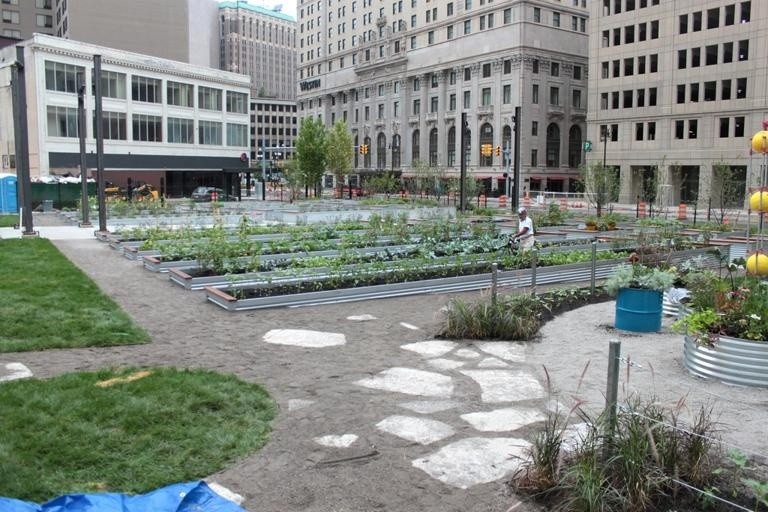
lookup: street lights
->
[599,125,611,216]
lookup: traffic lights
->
[360,144,364,155]
[487,144,492,157]
[496,146,501,156]
[481,144,486,155]
[364,145,368,155]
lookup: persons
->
[512,207,534,255]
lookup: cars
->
[191,187,236,203]
[259,174,281,183]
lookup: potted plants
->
[658,255,709,319]
[603,261,669,335]
[670,249,768,389]
[673,270,732,337]
